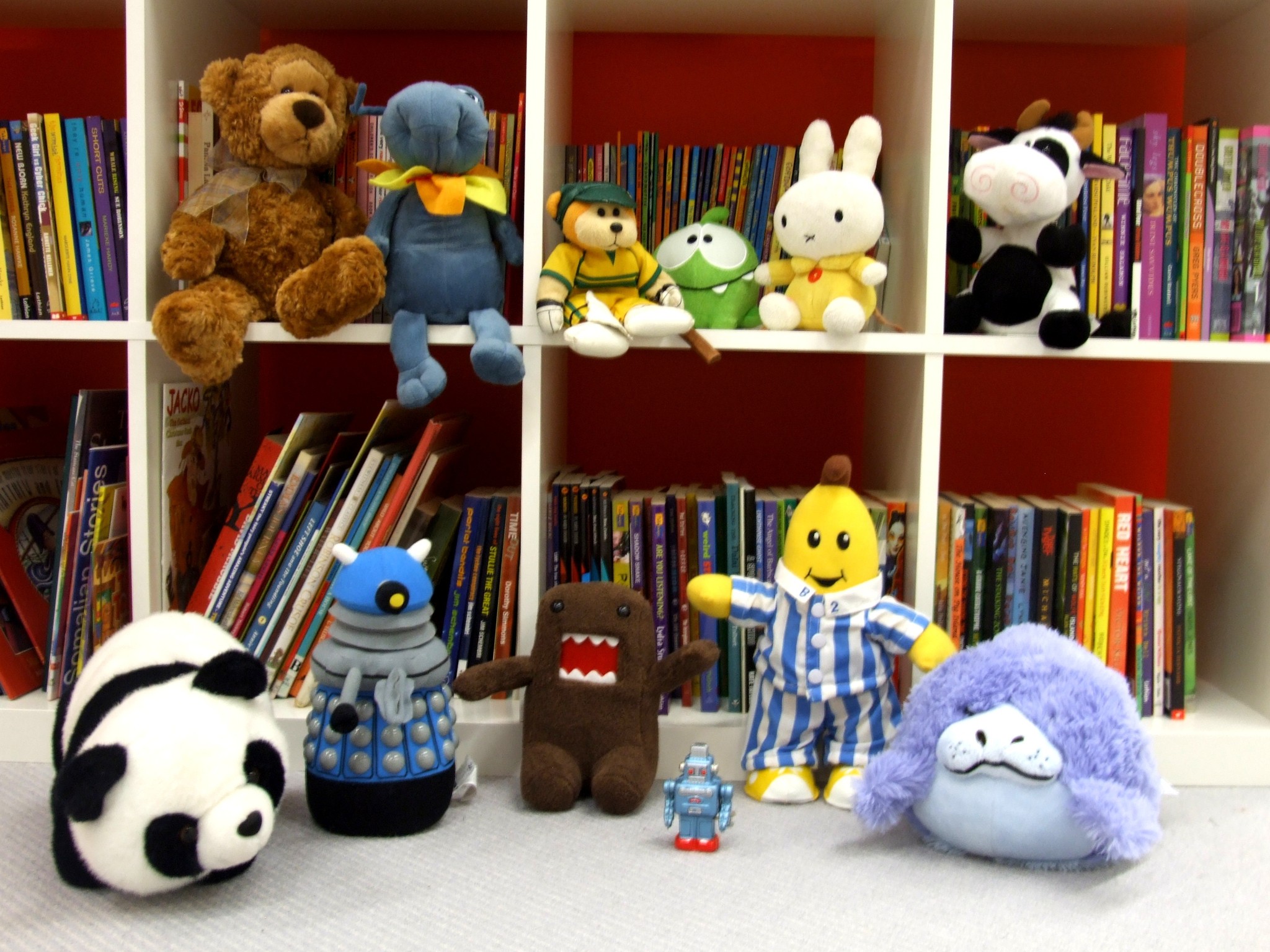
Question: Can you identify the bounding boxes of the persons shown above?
[1142,178,1163,218]
[662,742,733,852]
[886,510,907,578]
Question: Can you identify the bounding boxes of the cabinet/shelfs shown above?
[0,1,1270,792]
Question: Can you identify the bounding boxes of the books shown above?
[949,114,1270,341]
[0,112,127,321]
[0,386,129,700]
[177,80,525,328]
[160,379,519,707]
[936,482,1195,720]
[545,467,906,715]
[564,131,891,332]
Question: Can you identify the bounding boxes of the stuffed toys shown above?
[652,206,761,328]
[754,115,887,333]
[50,610,287,897]
[945,100,1126,350]
[854,623,1170,867]
[685,456,957,809]
[303,538,459,836]
[152,45,388,390]
[451,582,720,813]
[350,81,526,410]
[535,181,695,358]
